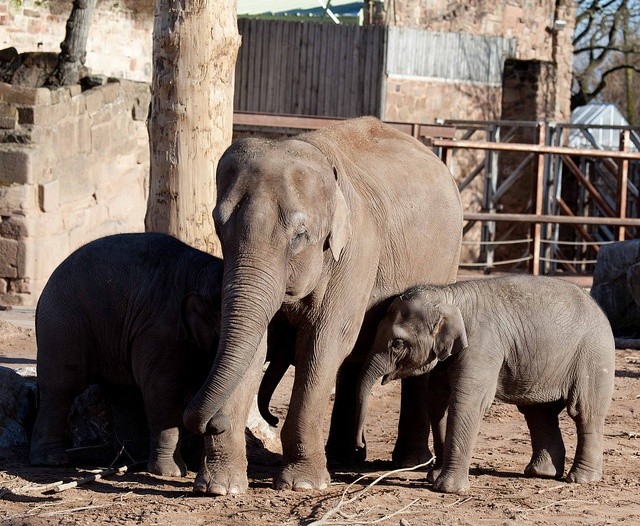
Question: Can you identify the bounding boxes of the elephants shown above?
[181,114,464,497]
[350,272,617,496]
[28,231,302,479]
